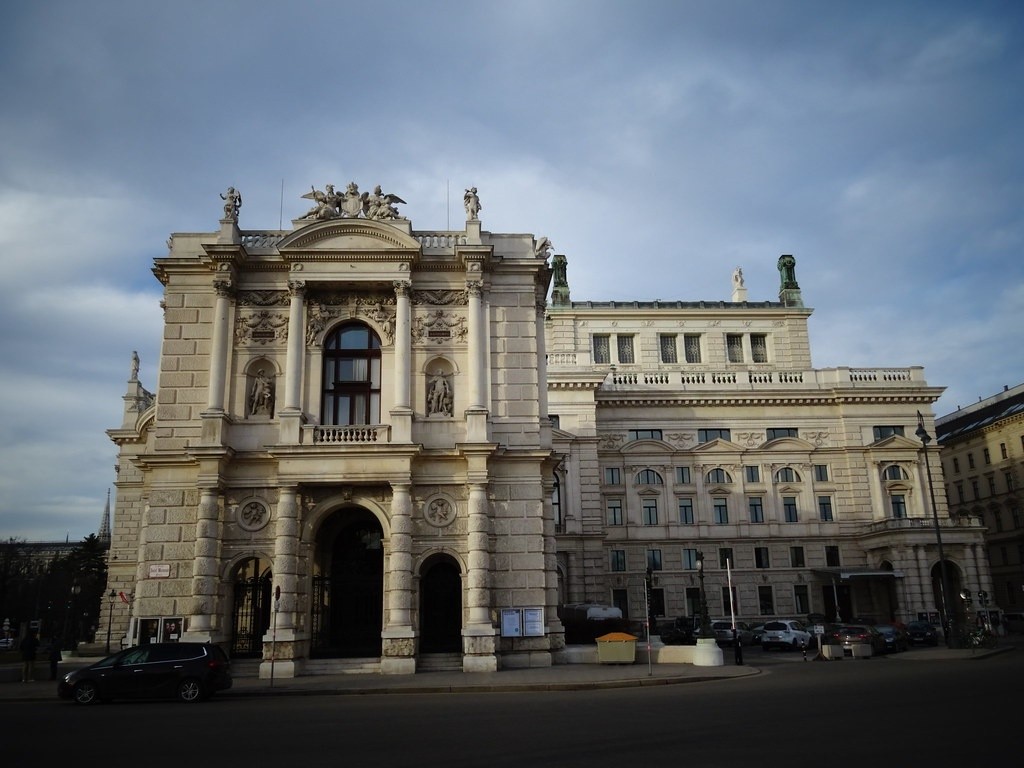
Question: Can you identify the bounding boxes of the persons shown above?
[249,369,271,414]
[428,370,449,413]
[314,185,349,209]
[463,187,482,220]
[131,351,139,382]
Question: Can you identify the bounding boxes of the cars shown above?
[873,625,908,652]
[57,641,232,705]
[0,638,16,650]
[710,621,755,644]
[831,625,890,655]
[903,621,940,646]
[761,620,814,652]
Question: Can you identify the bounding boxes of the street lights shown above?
[106,589,117,655]
[915,408,950,646]
[645,568,654,635]
[72,577,82,640]
[695,551,714,638]
